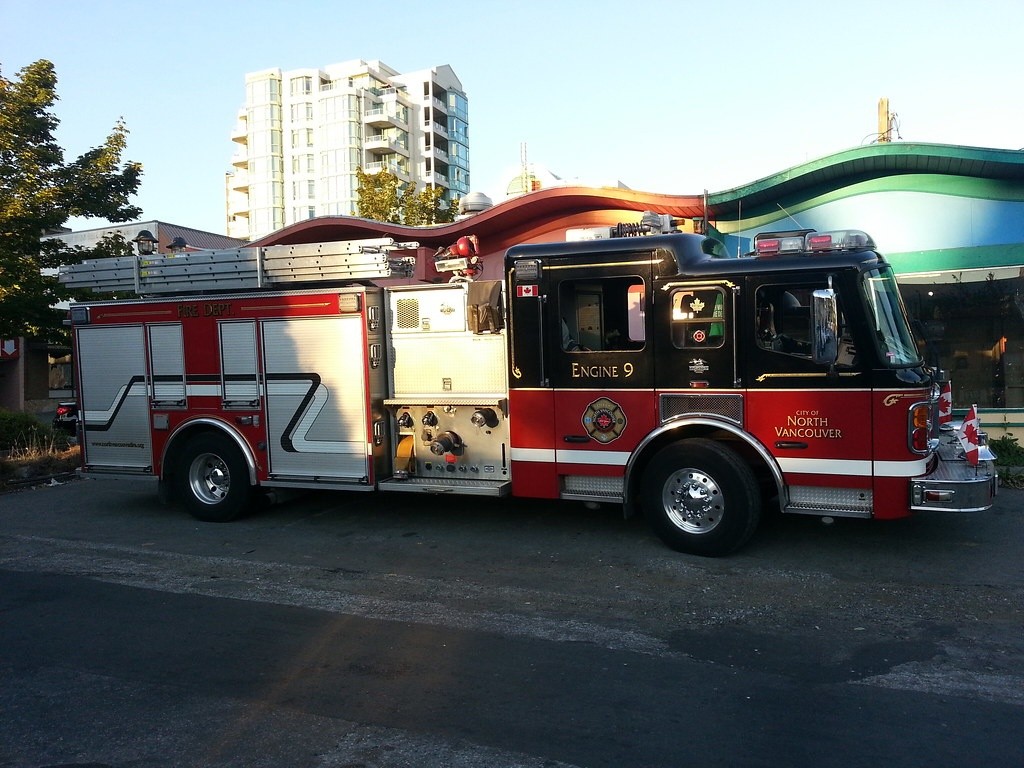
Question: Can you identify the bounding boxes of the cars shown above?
[52,401,78,438]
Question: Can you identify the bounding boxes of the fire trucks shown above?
[58,209,1001,560]
